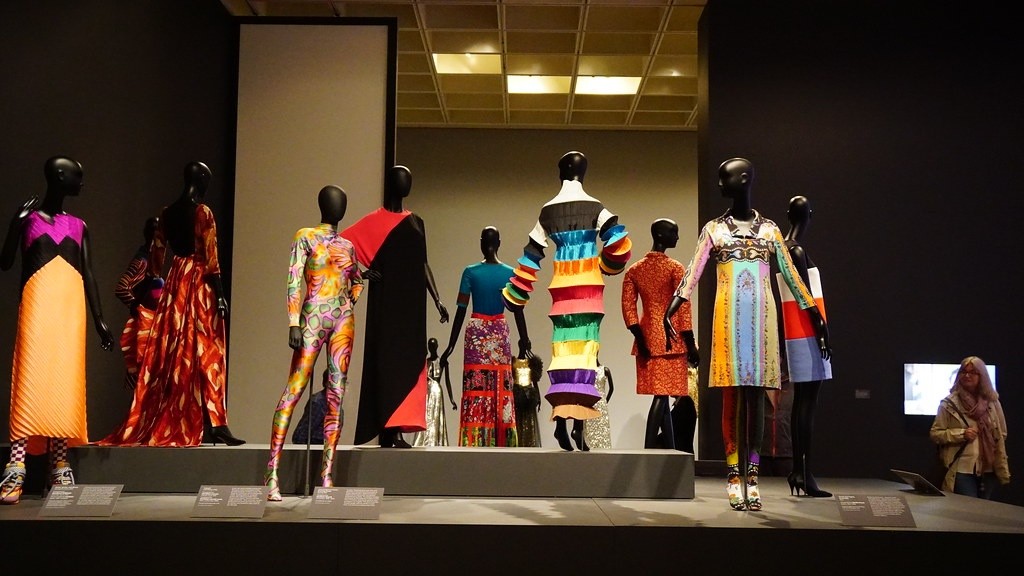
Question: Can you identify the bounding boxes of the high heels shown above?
[727,471,744,511]
[795,474,833,497]
[211,426,246,446]
[787,472,796,495]
[746,469,762,511]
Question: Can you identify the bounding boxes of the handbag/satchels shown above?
[935,466,948,491]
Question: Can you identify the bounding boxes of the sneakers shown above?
[47,463,75,491]
[0,463,26,505]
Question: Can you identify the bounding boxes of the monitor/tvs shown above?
[904,364,996,416]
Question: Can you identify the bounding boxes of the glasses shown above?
[959,370,980,376]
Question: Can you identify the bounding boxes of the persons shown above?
[263,186,364,504]
[511,338,542,448]
[292,368,344,446]
[770,195,832,497]
[930,356,1011,500]
[503,150,632,453]
[622,219,698,450]
[0,156,113,505]
[412,337,457,447]
[438,227,530,448]
[337,166,449,448]
[96,162,247,449]
[665,157,835,512]
